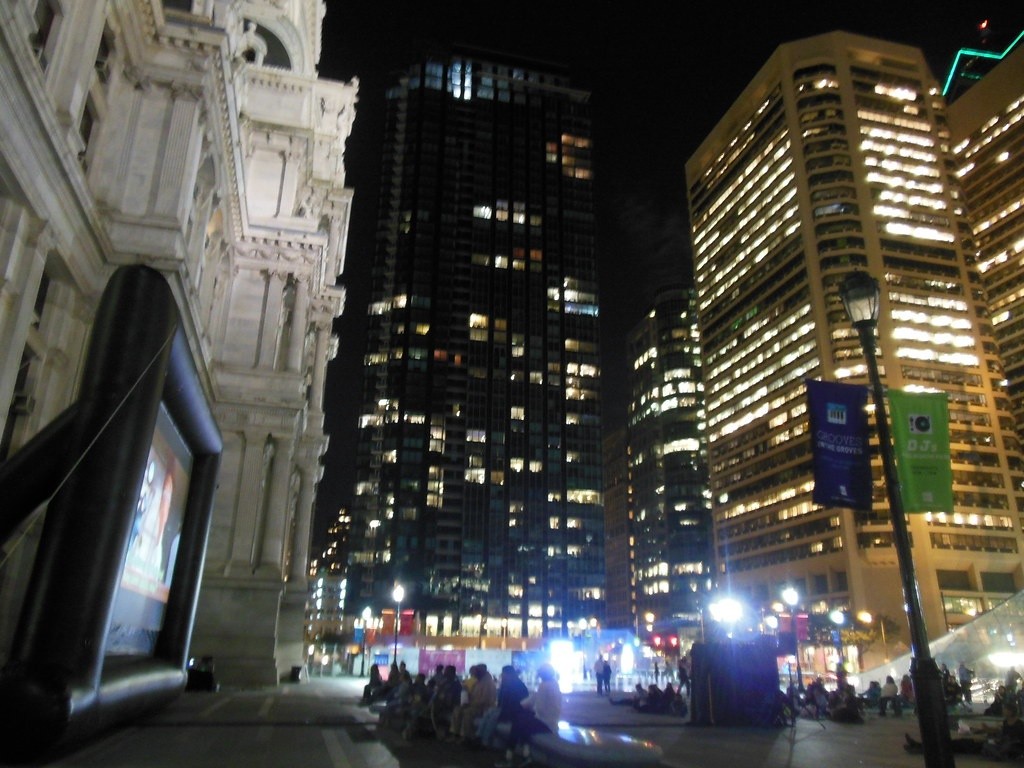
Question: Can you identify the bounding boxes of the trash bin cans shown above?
[290,666,302,685]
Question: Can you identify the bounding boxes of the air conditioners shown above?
[33,47,49,72]
[93,59,111,85]
[78,155,89,175]
[10,393,37,416]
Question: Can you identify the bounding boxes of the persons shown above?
[901,733,1009,753]
[984,686,1024,718]
[608,657,689,718]
[380,664,560,767]
[997,703,1024,759]
[603,661,612,693]
[788,661,975,720]
[879,674,901,716]
[360,664,381,704]
[595,656,604,695]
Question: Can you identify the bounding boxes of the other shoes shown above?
[518,757,531,768]
[456,735,472,746]
[494,759,512,767]
[471,744,486,751]
[905,734,920,749]
[469,737,481,744]
[904,744,917,755]
[443,732,460,744]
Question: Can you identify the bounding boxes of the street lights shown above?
[836,266,959,768]
[391,585,405,664]
[360,607,372,679]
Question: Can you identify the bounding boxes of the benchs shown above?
[368,702,665,768]
